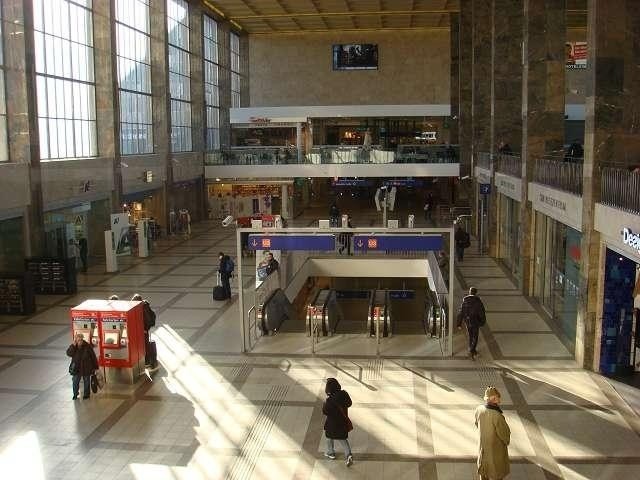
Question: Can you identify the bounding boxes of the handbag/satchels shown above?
[345,417,353,432]
[91,368,104,393]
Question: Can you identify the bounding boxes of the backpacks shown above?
[225,258,234,273]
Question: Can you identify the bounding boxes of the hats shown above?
[484,387,498,396]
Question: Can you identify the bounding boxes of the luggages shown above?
[147,329,157,364]
[213,270,225,300]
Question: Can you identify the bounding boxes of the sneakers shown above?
[346,455,352,467]
[325,452,335,459]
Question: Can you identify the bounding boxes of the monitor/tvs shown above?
[104,332,118,345]
[331,43,379,71]
[77,333,89,342]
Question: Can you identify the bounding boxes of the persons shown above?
[565,139,585,163]
[565,44,576,66]
[258,250,270,281]
[329,205,340,227]
[387,138,458,163]
[76,233,89,271]
[65,334,100,400]
[338,216,353,256]
[474,387,511,480]
[131,294,150,367]
[457,286,485,362]
[498,141,514,156]
[454,228,464,262]
[217,252,232,299]
[423,193,433,220]
[322,377,353,467]
[67,240,77,268]
[266,252,279,277]
[241,216,290,259]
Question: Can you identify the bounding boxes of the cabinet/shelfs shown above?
[0,271,37,316]
[23,257,77,296]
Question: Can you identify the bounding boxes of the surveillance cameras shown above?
[221,215,233,227]
